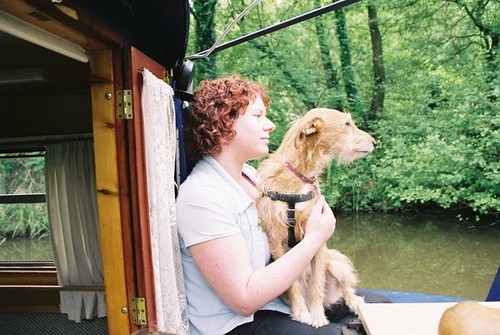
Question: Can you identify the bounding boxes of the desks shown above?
[356,299,500,335]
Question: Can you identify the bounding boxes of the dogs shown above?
[254,106,377,330]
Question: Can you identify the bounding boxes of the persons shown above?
[174,75,367,335]
